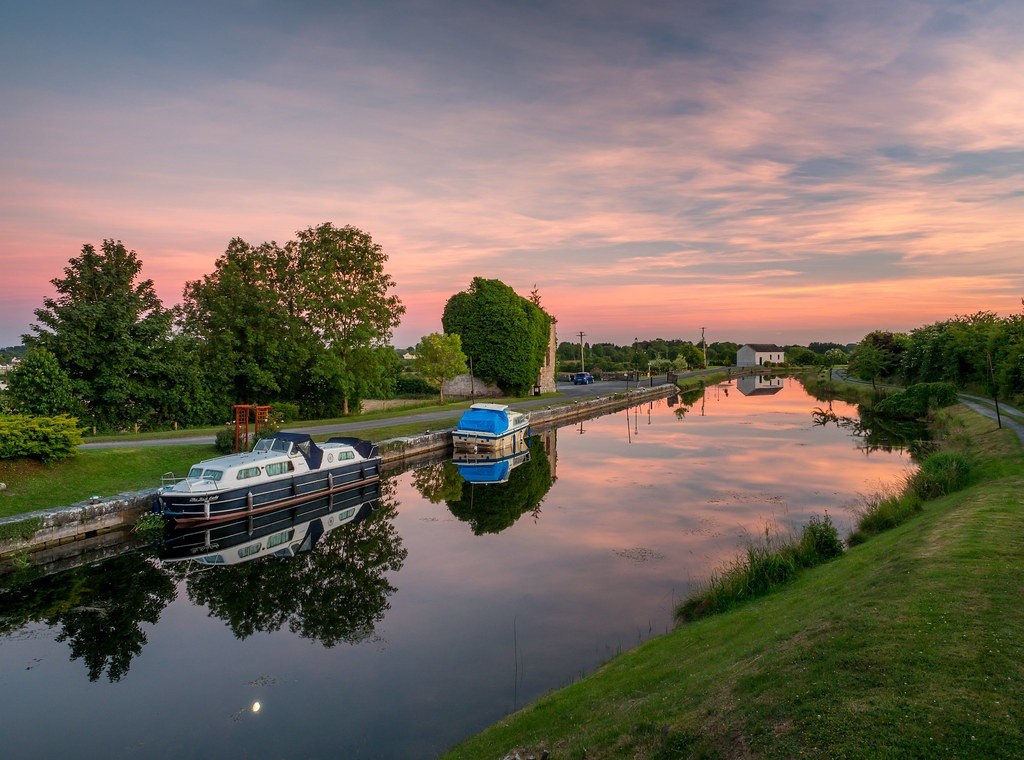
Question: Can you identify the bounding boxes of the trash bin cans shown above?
[533,385,541,396]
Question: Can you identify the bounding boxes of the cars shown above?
[573,372,594,385]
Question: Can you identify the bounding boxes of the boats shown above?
[451,403,531,451]
[155,480,382,578]
[157,432,382,524]
[451,439,532,485]
